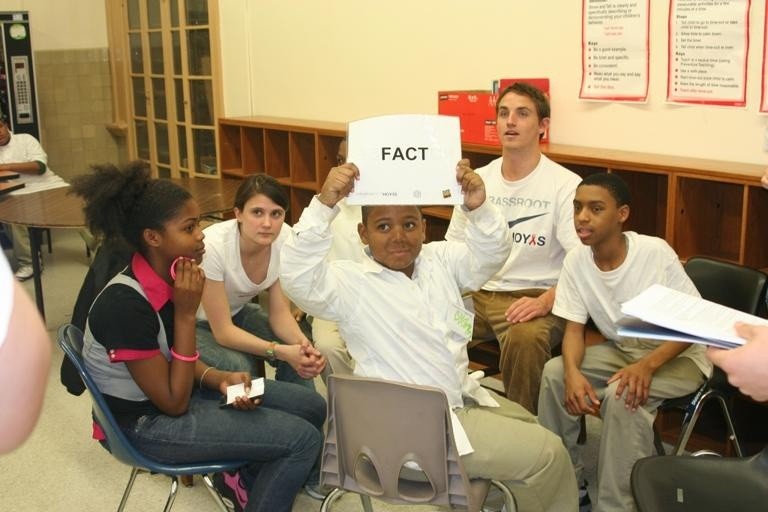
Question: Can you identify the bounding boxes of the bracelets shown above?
[265,340,278,362]
[197,365,216,392]
[169,346,200,363]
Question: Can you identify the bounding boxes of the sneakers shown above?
[305,478,343,501]
[212,468,250,511]
[14,265,41,281]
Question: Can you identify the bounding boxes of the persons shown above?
[274,159,582,510]
[1,245,53,457]
[535,172,715,510]
[438,81,583,421]
[184,172,339,502]
[0,119,102,283]
[66,159,328,512]
[706,321,768,404]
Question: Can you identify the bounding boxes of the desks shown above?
[0,173,245,330]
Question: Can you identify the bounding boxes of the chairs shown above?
[318,374,517,512]
[593,258,768,458]
[58,322,250,512]
[469,338,587,445]
[629,455,767,512]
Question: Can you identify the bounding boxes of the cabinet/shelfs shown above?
[213,112,767,460]
